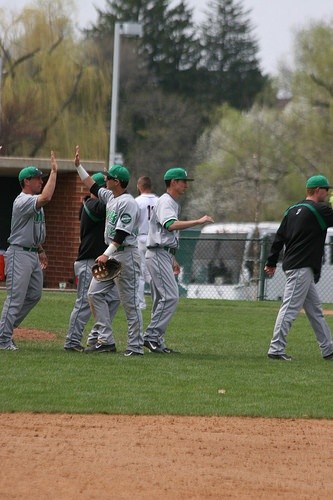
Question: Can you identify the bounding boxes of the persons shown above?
[72,145,147,357]
[265,174,333,362]
[140,168,215,352]
[62,172,121,352]
[0,151,58,351]
[127,173,168,308]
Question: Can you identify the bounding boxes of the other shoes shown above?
[2,343,18,350]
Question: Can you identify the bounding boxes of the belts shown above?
[164,247,175,255]
[23,247,38,252]
[117,246,124,251]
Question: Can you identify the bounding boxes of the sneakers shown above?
[68,345,85,352]
[163,348,177,354]
[268,353,292,361]
[84,342,115,353]
[118,350,142,357]
[324,353,333,359]
[143,340,163,353]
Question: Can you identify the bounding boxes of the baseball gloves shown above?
[91,258,122,282]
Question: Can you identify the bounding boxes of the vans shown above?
[185,221,333,304]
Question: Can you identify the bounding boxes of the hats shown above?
[18,166,48,181]
[305,175,333,188]
[164,168,195,181]
[91,173,106,186]
[104,165,129,180]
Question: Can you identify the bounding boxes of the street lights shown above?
[108,20,143,168]
